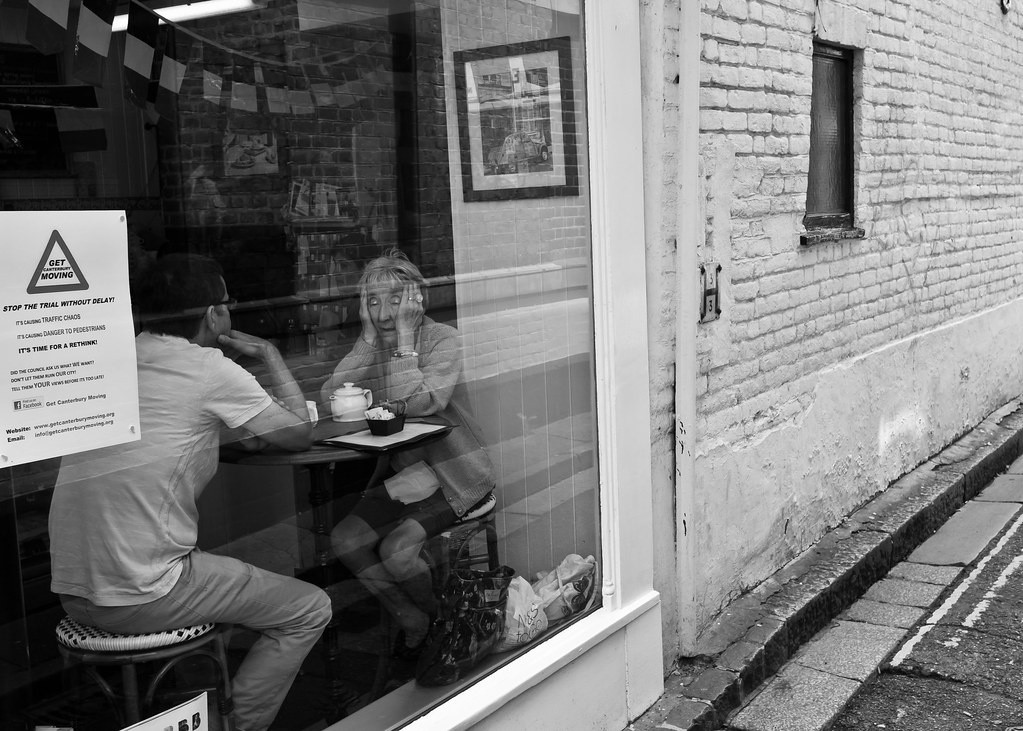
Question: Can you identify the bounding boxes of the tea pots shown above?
[329,382,373,423]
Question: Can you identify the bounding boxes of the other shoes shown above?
[386,611,436,681]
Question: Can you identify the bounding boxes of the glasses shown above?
[201,299,237,316]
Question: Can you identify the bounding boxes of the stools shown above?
[57,615,234,731]
[390,493,501,654]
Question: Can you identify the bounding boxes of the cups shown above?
[305,400,318,429]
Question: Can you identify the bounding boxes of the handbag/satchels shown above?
[493,575,549,654]
[530,554,600,628]
[415,524,517,687]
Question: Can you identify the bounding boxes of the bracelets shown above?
[395,350,419,357]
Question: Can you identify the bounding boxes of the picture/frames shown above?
[453,36,580,202]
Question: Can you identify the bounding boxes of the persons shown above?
[322,252,495,683]
[47,253,334,731]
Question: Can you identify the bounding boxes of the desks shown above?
[218,414,454,724]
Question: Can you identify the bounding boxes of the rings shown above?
[415,294,423,303]
[408,297,414,301]
